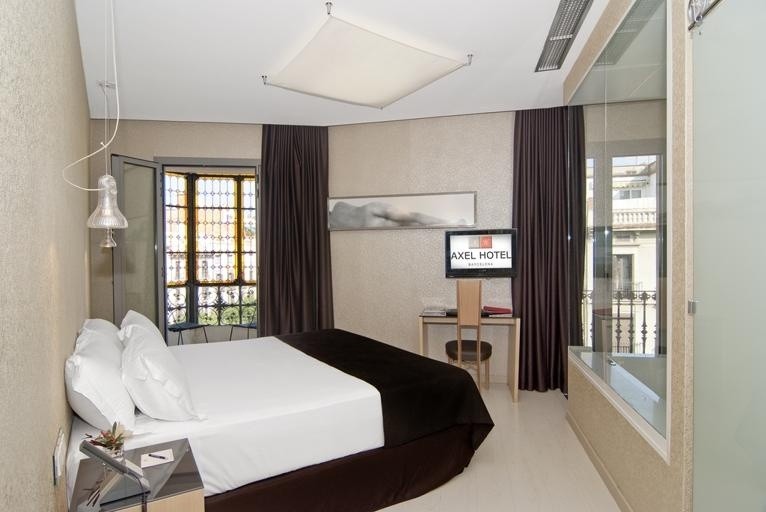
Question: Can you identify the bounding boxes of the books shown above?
[421,305,512,317]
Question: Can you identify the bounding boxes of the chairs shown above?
[168,305,209,345]
[446,281,492,393]
[229,306,257,340]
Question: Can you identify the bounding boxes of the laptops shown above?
[446,309,489,317]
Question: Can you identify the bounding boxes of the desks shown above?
[419,316,520,403]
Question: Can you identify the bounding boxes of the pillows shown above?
[64,309,205,432]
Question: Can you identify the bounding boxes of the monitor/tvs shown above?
[445,228,518,279]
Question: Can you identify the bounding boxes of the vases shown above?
[102,441,124,465]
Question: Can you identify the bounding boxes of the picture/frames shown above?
[327,191,477,231]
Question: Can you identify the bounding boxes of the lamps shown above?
[61,0,128,249]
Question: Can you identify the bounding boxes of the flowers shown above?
[83,422,134,454]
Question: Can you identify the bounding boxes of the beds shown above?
[67,328,495,512]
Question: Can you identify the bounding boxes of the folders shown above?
[484,307,511,314]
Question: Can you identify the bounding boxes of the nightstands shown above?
[69,438,205,512]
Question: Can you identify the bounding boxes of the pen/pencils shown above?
[149,454,165,459]
